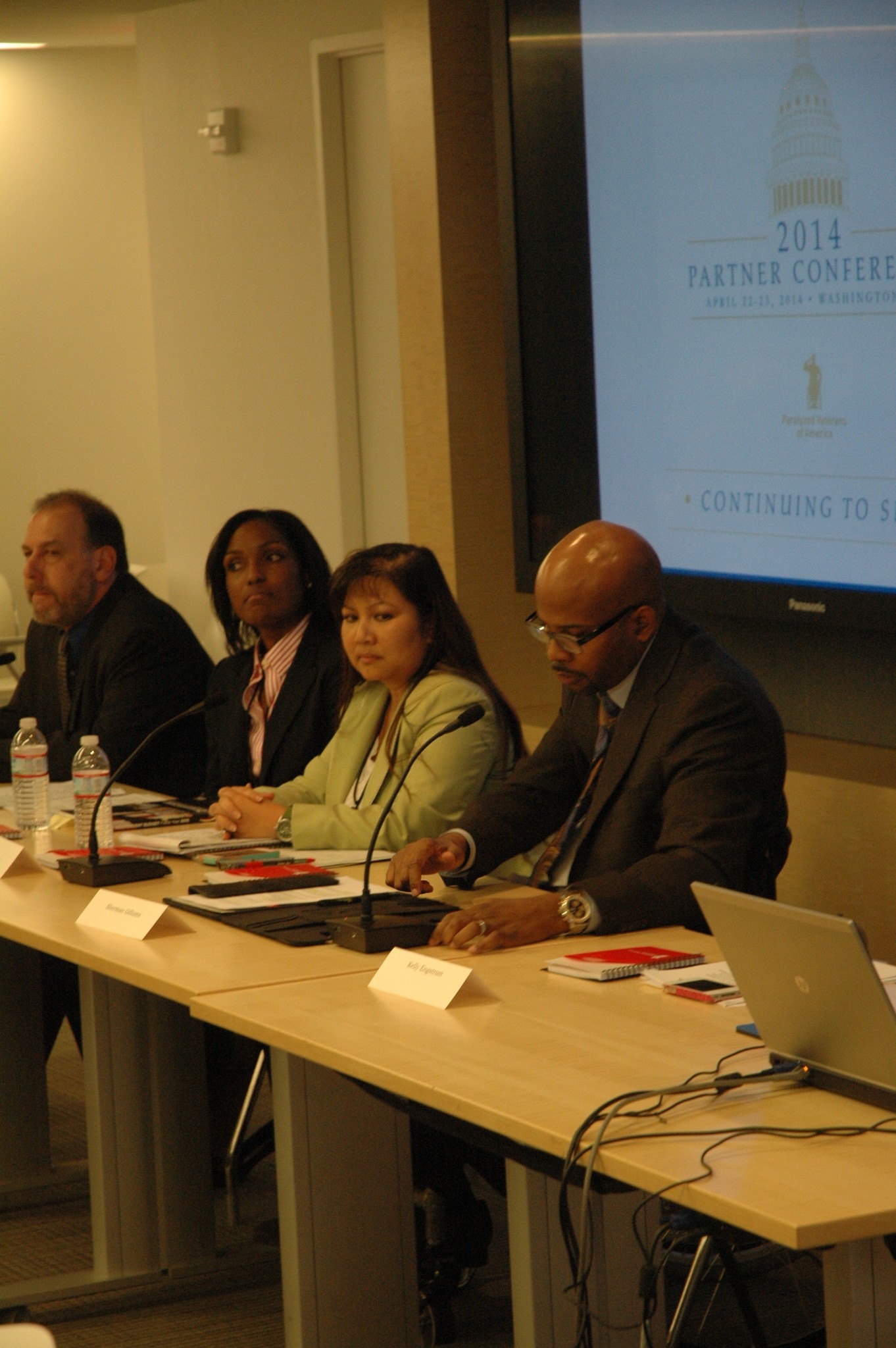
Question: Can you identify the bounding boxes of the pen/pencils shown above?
[235,857,315,868]
[206,852,280,865]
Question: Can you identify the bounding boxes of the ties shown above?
[529,692,622,890]
[57,633,73,732]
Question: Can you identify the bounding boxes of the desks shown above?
[0,780,896,1348]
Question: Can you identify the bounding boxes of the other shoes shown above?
[214,1119,274,1186]
[418,1199,494,1281]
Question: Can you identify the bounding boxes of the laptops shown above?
[689,879,896,1112]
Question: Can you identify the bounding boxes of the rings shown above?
[476,920,490,937]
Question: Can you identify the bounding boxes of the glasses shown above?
[522,598,653,654]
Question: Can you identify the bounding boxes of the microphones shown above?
[56,690,230,888]
[322,704,488,953]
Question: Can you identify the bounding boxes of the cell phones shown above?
[663,978,743,1004]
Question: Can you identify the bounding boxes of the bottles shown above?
[11,717,50,832]
[72,736,113,850]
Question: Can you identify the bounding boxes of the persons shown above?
[0,491,216,1063]
[193,510,793,1289]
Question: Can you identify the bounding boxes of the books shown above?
[546,946,706,982]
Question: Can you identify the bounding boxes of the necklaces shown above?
[353,697,397,810]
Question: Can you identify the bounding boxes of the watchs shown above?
[558,890,591,937]
[274,803,294,842]
